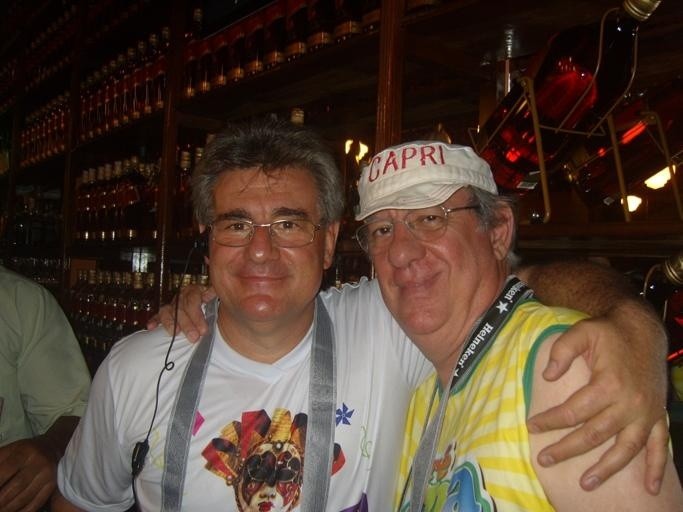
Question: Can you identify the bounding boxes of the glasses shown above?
[208,218,328,248]
[351,204,482,256]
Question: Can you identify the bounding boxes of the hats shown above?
[353,139,500,221]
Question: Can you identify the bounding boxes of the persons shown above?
[51,116,667,512]
[1,262,92,512]
[144,141,682,512]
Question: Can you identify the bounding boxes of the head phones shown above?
[191,224,210,258]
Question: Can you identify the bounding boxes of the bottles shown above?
[629,255,682,409]
[0,1,450,358]
[455,1,663,212]
